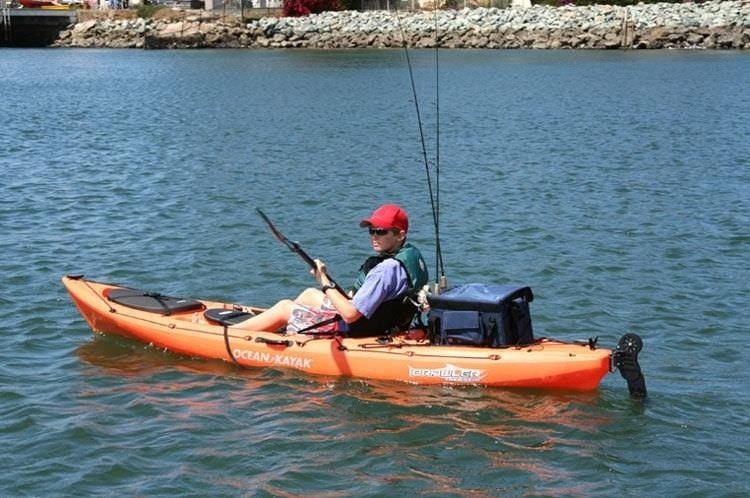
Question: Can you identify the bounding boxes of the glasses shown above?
[368,227,399,236]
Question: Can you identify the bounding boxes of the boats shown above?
[0,0,81,51]
[58,266,650,401]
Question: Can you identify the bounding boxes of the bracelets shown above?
[321,281,337,294]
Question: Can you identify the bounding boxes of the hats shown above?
[359,204,409,234]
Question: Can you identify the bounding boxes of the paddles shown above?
[257,208,348,297]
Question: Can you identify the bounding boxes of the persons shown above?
[190,204,431,337]
